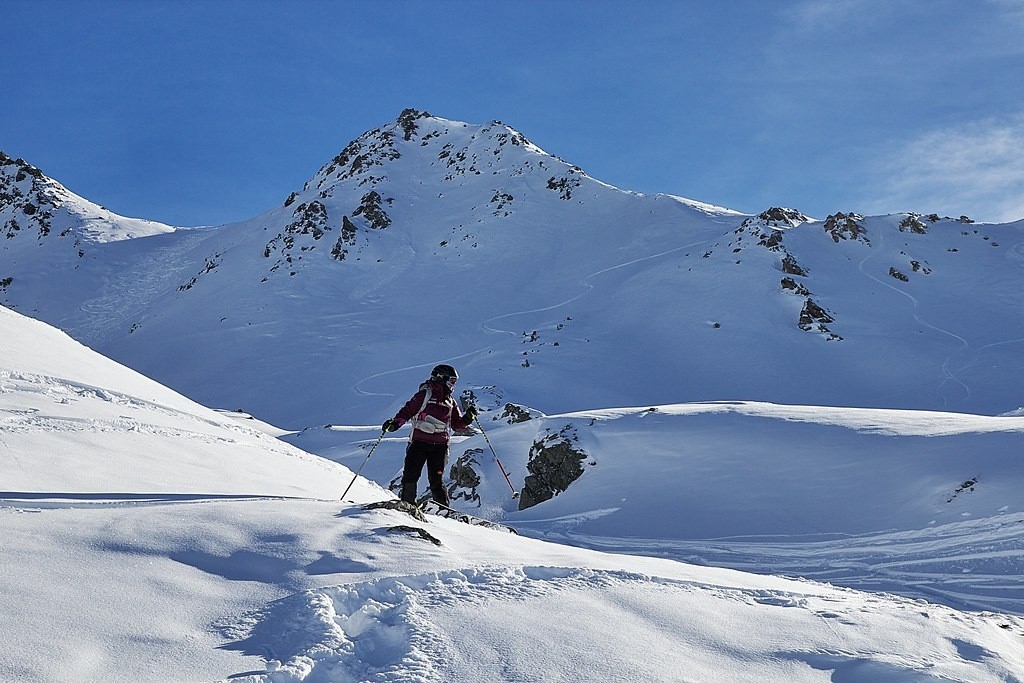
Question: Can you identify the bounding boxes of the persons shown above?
[381,364,479,507]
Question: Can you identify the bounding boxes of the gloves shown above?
[382,417,399,433]
[461,405,477,425]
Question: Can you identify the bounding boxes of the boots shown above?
[400,482,416,506]
[430,482,450,509]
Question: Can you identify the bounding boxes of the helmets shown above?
[430,364,459,379]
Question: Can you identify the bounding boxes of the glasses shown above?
[447,377,457,384]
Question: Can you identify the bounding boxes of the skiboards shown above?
[417,500,518,536]
